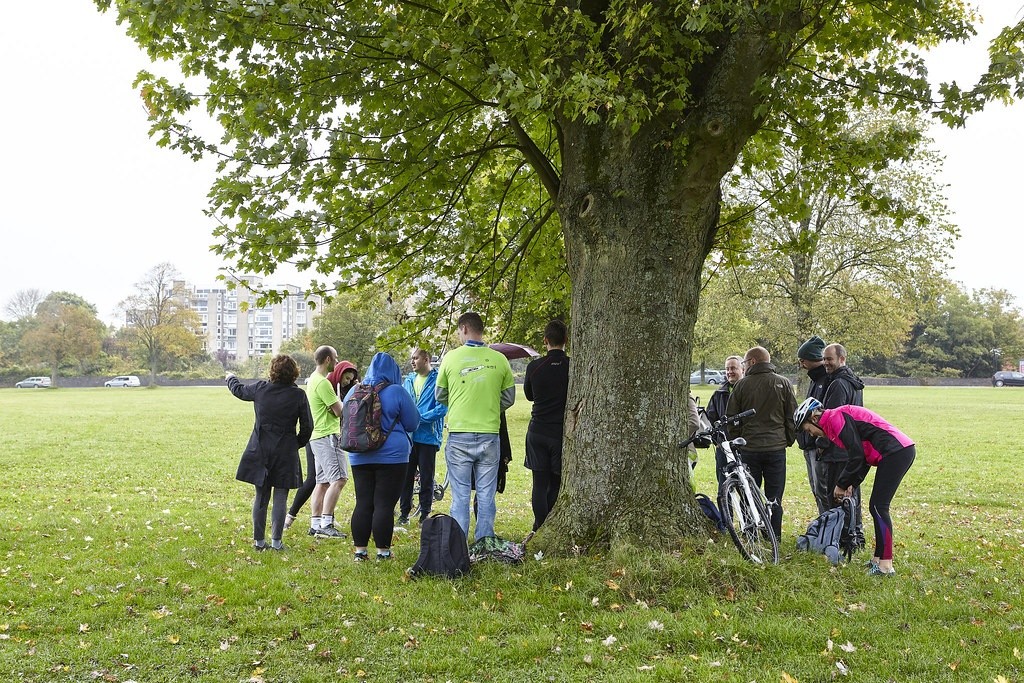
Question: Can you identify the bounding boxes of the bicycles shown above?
[393,426,449,520]
[679,409,779,568]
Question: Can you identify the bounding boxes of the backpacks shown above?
[689,396,713,448]
[409,513,471,583]
[797,496,857,567]
[339,381,400,452]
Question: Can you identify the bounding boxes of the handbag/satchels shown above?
[695,493,727,536]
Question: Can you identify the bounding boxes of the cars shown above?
[719,370,728,383]
[690,370,724,384]
[992,371,1024,387]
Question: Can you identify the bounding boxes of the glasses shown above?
[740,358,753,367]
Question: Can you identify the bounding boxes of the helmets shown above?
[793,396,823,430]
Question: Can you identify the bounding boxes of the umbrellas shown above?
[488,343,541,360]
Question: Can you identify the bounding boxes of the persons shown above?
[225,353,315,553]
[284,346,359,540]
[398,349,449,526]
[706,355,747,532]
[523,320,575,531]
[342,352,422,563]
[795,336,866,553]
[723,346,800,546]
[686,394,697,479]
[434,313,515,545]
[793,396,916,578]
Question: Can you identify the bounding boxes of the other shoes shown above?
[417,515,430,527]
[334,520,345,528]
[396,514,410,526]
[282,522,290,531]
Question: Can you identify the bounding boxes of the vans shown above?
[104,376,140,387]
[15,377,51,388]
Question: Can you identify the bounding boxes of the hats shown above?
[797,335,827,361]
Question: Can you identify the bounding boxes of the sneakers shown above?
[354,553,369,561]
[255,542,271,550]
[867,559,874,567]
[315,524,347,539]
[868,565,896,577]
[270,542,284,551]
[376,550,393,562]
[306,527,315,538]
